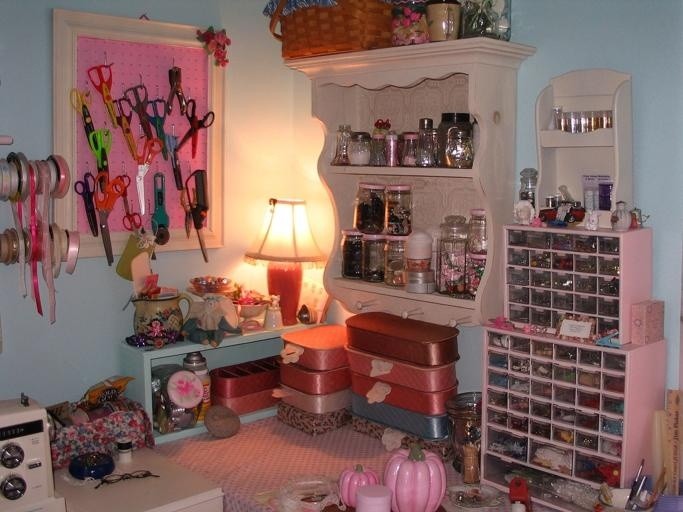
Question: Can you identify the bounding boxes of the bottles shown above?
[331,113,488,298]
[551,105,612,133]
[151,352,210,435]
[391,1,511,47]
[519,168,642,232]
[459,457,480,487]
[264,307,283,331]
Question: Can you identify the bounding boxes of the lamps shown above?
[245,198,326,325]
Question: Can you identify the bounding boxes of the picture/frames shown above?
[53,10,225,259]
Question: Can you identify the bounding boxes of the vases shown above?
[284,36,536,329]
[388,5,428,44]
[425,3,460,41]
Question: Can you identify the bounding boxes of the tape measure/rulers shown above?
[667,389,683,496]
[653,410,668,496]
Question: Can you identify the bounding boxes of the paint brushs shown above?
[601,459,668,511]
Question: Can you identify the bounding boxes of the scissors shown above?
[70,63,215,267]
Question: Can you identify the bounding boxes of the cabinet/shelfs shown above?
[122,324,310,446]
[479,68,667,512]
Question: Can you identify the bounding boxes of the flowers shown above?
[194,25,230,67]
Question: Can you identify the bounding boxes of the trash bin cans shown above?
[444,392,482,473]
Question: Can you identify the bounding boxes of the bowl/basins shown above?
[190,276,232,293]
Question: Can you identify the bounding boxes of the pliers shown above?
[165,66,187,115]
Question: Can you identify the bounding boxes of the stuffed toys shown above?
[177,291,244,347]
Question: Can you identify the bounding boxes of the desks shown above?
[54,447,226,512]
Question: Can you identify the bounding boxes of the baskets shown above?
[280,0,392,59]
